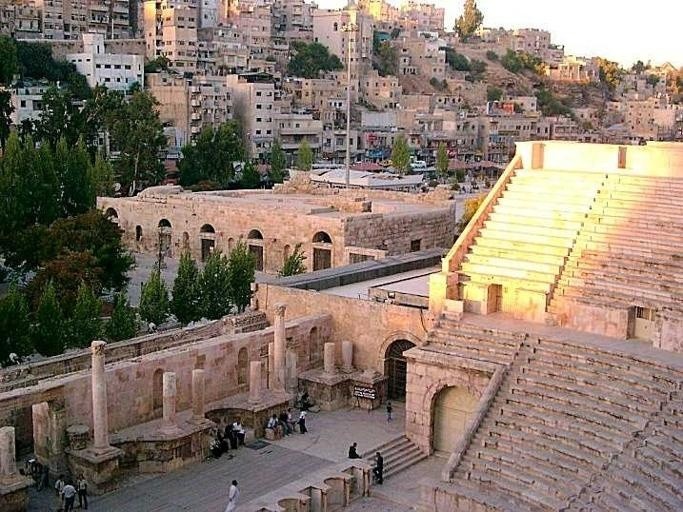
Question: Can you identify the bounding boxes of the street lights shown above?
[339,23,359,187]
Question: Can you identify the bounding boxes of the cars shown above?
[410,160,426,169]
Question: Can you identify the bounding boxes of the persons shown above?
[222,480,240,512]
[371,452,383,485]
[53,474,65,512]
[385,402,392,423]
[75,474,88,510]
[58,481,77,512]
[348,442,362,460]
[208,407,307,460]
